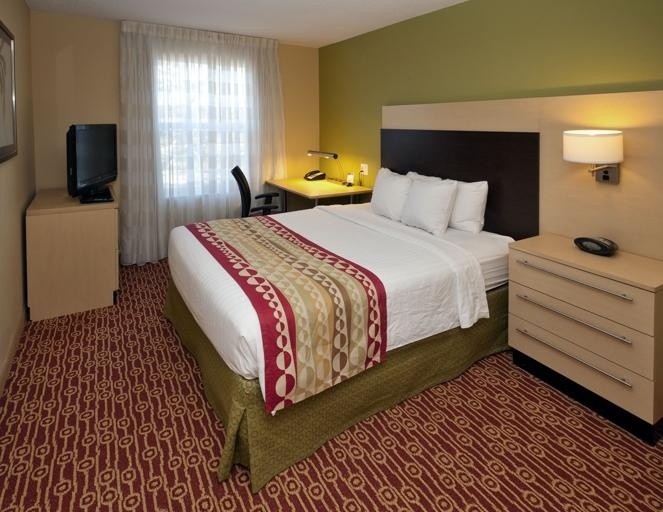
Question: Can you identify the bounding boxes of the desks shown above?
[265,176,373,211]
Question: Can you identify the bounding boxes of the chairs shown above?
[229,166,284,218]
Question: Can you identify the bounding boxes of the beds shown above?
[157,128,541,493]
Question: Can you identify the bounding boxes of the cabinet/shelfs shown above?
[23,184,119,321]
[507,230,663,446]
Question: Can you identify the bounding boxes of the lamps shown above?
[561,129,624,185]
[304,149,346,185]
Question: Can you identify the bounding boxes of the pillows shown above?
[442,179,488,234]
[367,167,413,221]
[399,179,458,237]
[404,170,442,182]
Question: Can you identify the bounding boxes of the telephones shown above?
[304,170,326,181]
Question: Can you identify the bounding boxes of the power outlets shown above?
[360,164,368,176]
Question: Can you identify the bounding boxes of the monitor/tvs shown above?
[66,124,117,203]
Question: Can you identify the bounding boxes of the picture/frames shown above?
[0,21,19,163]
